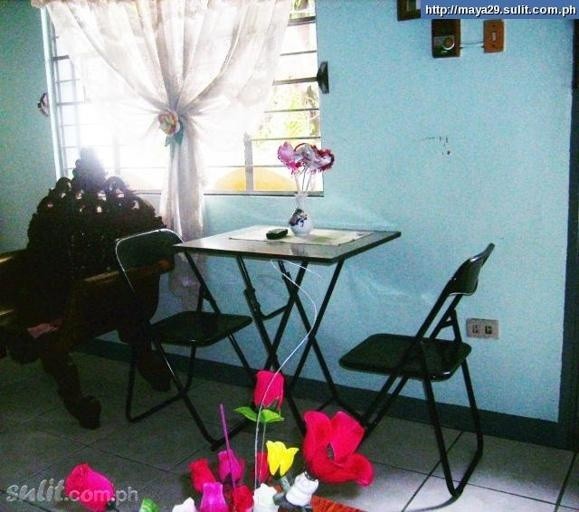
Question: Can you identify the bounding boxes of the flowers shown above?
[278,142,335,195]
[64,368,373,511]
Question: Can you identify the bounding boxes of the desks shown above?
[172,225,401,456]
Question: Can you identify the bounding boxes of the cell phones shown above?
[266,228,288,239]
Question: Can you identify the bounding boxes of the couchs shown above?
[1,148,183,429]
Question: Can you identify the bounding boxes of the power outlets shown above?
[466,318,498,339]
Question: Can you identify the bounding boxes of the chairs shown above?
[338,242,496,499]
[114,229,258,451]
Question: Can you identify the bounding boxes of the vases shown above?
[290,195,313,238]
